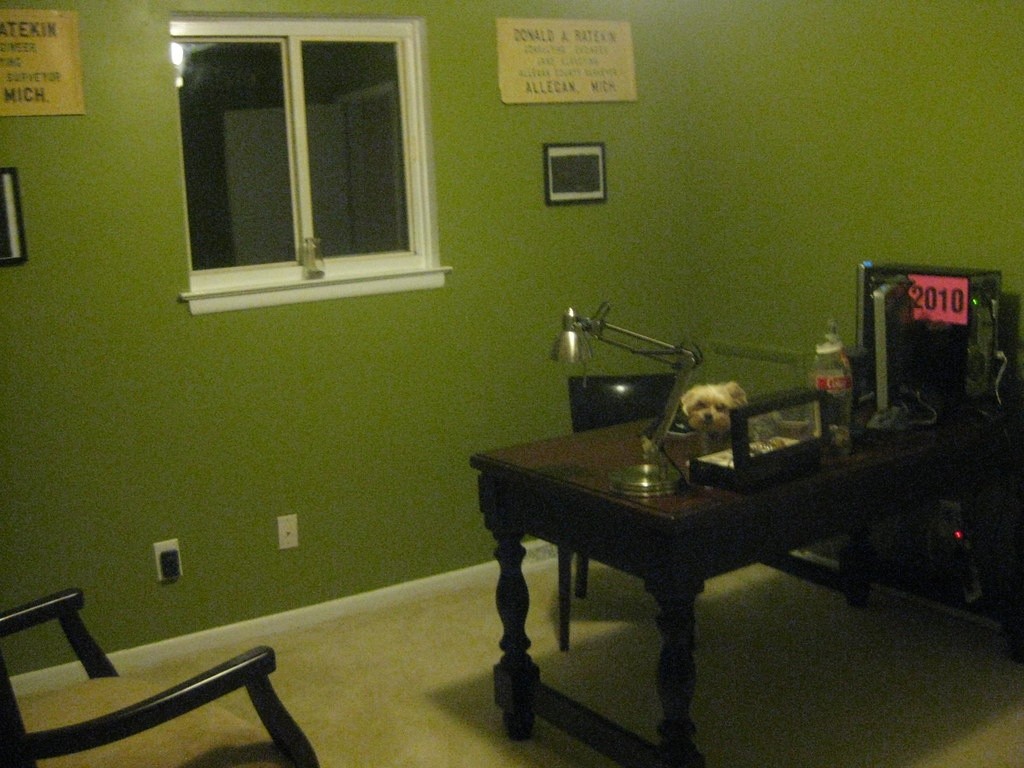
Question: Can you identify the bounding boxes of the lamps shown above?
[551,301,696,497]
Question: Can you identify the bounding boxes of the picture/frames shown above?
[0,167,28,267]
[545,142,607,205]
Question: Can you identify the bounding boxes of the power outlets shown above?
[153,538,184,582]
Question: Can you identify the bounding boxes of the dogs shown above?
[681,381,812,444]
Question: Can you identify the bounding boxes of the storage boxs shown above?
[690,388,830,494]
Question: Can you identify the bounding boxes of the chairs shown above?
[559,373,705,652]
[0,588,320,768]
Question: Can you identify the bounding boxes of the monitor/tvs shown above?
[864,275,944,431]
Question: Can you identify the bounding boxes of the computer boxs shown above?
[857,263,999,404]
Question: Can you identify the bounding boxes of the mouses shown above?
[652,415,696,439]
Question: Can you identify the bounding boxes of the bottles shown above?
[810,323,853,453]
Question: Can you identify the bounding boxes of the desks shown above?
[469,388,1024,768]
